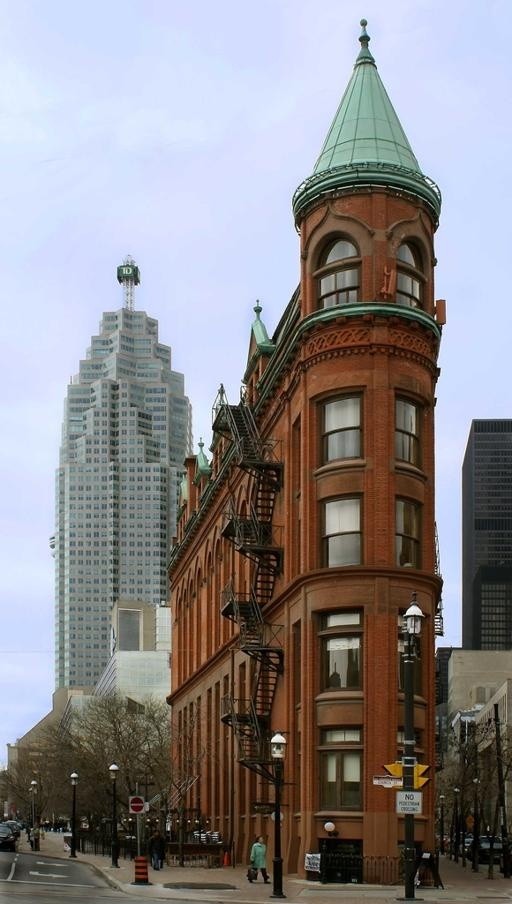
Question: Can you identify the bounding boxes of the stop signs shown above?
[129,797,145,813]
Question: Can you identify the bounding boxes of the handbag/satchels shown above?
[247,869,258,880]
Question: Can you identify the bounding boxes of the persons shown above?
[443,838,450,852]
[26,818,64,851]
[148,829,164,870]
[250,836,271,884]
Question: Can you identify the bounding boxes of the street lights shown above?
[108,765,120,866]
[439,795,446,855]
[70,771,78,857]
[28,781,38,829]
[472,778,482,872]
[403,595,425,898]
[269,733,286,898]
[453,788,460,862]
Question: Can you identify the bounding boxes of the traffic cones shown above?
[223,852,230,866]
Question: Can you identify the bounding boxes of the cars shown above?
[436,832,512,874]
[0,820,23,853]
